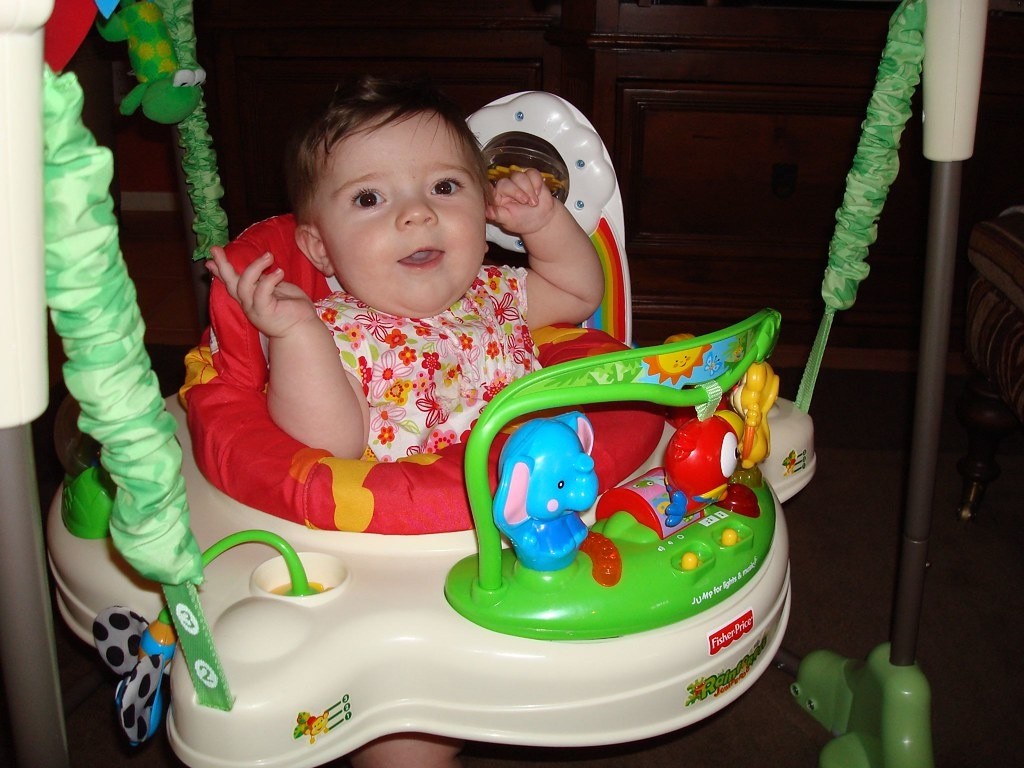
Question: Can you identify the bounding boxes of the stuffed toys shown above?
[95,0,205,122]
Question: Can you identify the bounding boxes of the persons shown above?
[206,78,606,768]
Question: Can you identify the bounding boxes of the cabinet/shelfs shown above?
[194,0,1024,378]
